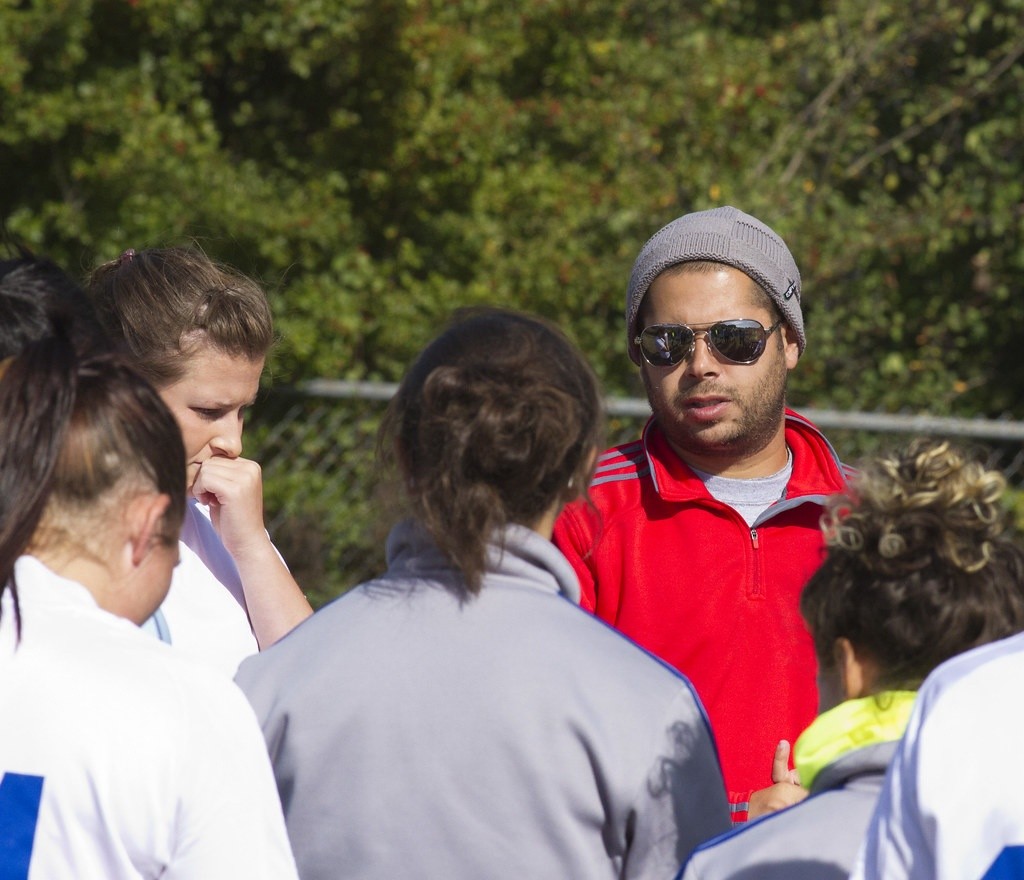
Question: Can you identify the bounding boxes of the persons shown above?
[544,205,883,832]
[228,305,734,880]
[1,255,111,369]
[0,337,299,880]
[81,243,318,682]
[850,630,1023,880]
[667,433,1022,880]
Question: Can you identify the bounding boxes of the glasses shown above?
[634,319,783,369]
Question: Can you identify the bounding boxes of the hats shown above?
[626,205,806,366]
